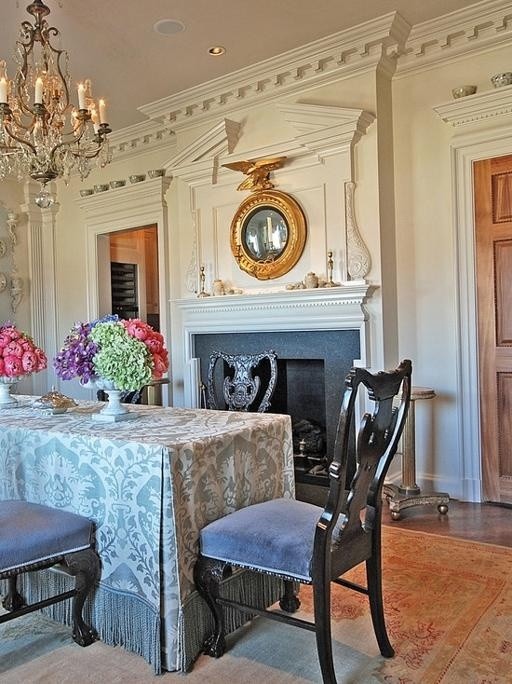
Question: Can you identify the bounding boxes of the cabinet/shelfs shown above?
[109,260,137,320]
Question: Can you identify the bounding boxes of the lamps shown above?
[1,2,113,208]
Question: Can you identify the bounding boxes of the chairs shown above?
[196,361,414,678]
[3,499,99,654]
[204,350,280,413]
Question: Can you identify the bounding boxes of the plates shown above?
[77,400,105,414]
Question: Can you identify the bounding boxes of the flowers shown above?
[0,312,169,393]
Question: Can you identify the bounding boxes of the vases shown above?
[80,375,144,421]
[1,374,27,408]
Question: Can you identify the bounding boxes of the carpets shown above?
[1,521,511,681]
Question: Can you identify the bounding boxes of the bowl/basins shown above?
[451,85,477,99]
[490,72,512,89]
[79,169,165,197]
